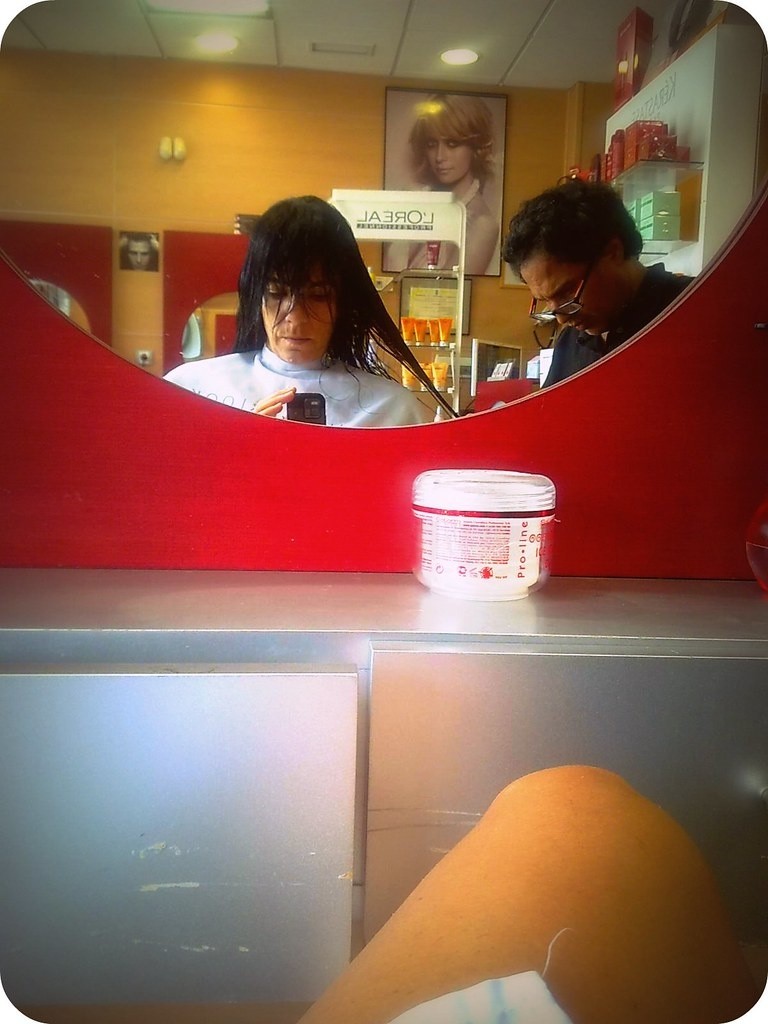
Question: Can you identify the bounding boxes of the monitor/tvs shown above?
[471,338,522,396]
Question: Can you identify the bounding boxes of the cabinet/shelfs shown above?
[605,22,762,273]
[329,185,470,418]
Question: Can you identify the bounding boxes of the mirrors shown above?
[0,0,768,431]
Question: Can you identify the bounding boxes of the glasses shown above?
[528,260,603,321]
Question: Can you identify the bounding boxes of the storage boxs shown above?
[567,0,756,259]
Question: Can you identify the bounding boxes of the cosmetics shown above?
[394,315,453,392]
[408,470,558,601]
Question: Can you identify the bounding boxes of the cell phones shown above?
[286,392,327,426]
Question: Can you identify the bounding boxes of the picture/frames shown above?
[380,85,529,339]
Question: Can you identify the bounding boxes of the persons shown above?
[383,93,501,274]
[501,175,695,389]
[163,195,424,427]
[298,763,758,1024]
[119,233,159,271]
